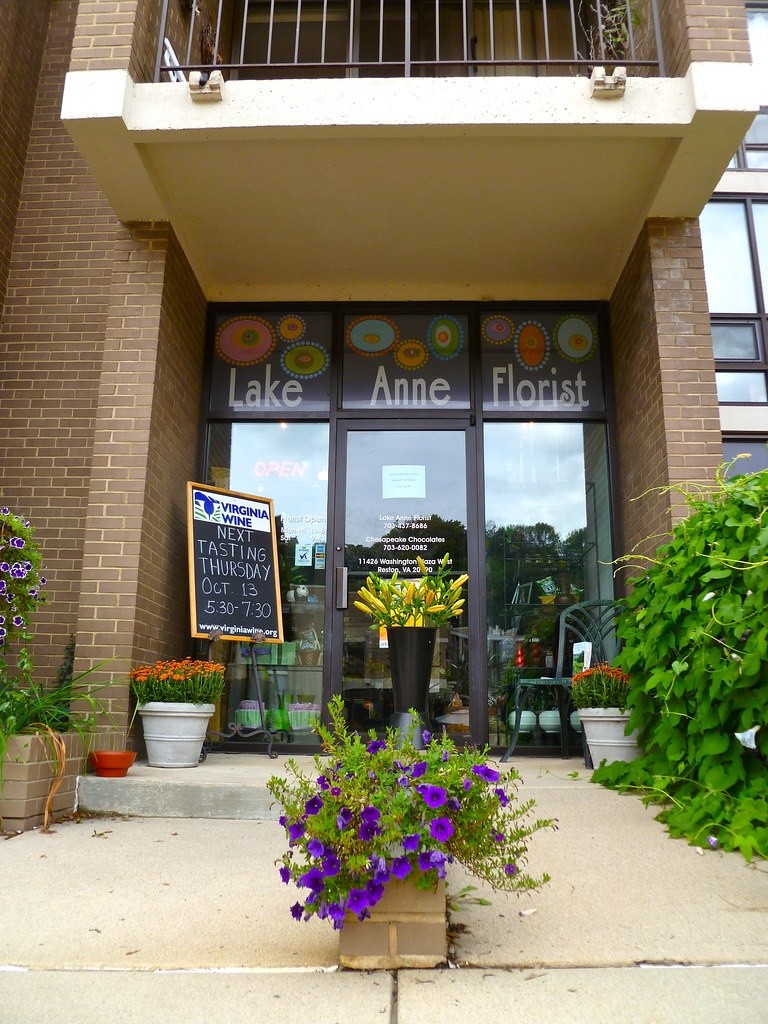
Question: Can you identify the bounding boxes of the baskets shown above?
[296,626,323,666]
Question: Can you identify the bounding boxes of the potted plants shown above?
[507,688,538,733]
[0,654,132,836]
[536,685,561,734]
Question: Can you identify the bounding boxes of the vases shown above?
[137,701,217,769]
[383,625,442,750]
[570,711,583,733]
[578,705,646,771]
[335,856,449,971]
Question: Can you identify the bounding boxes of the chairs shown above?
[498,598,630,771]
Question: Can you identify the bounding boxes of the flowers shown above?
[263,692,559,933]
[0,507,52,648]
[128,656,227,706]
[566,660,634,708]
[353,551,469,629]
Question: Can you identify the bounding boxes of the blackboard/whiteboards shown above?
[184,480,285,646]
